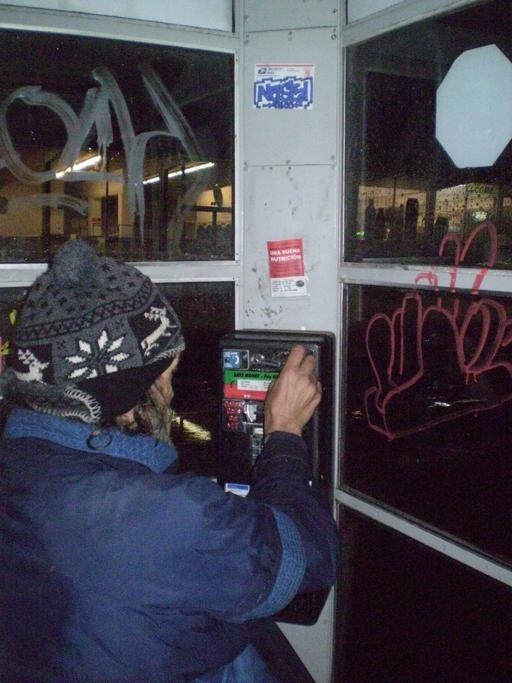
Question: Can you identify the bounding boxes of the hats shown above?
[10,238,187,424]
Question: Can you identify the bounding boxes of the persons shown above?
[0,235,342,683]
